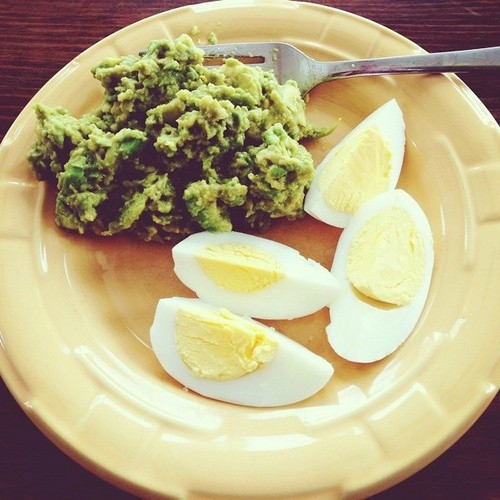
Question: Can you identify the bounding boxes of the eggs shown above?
[324,184,436,367]
[171,227,341,322]
[302,97,409,229]
[150,296,336,412]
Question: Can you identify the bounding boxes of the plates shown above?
[0,0,499,500]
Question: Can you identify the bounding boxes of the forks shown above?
[190,39,499,100]
